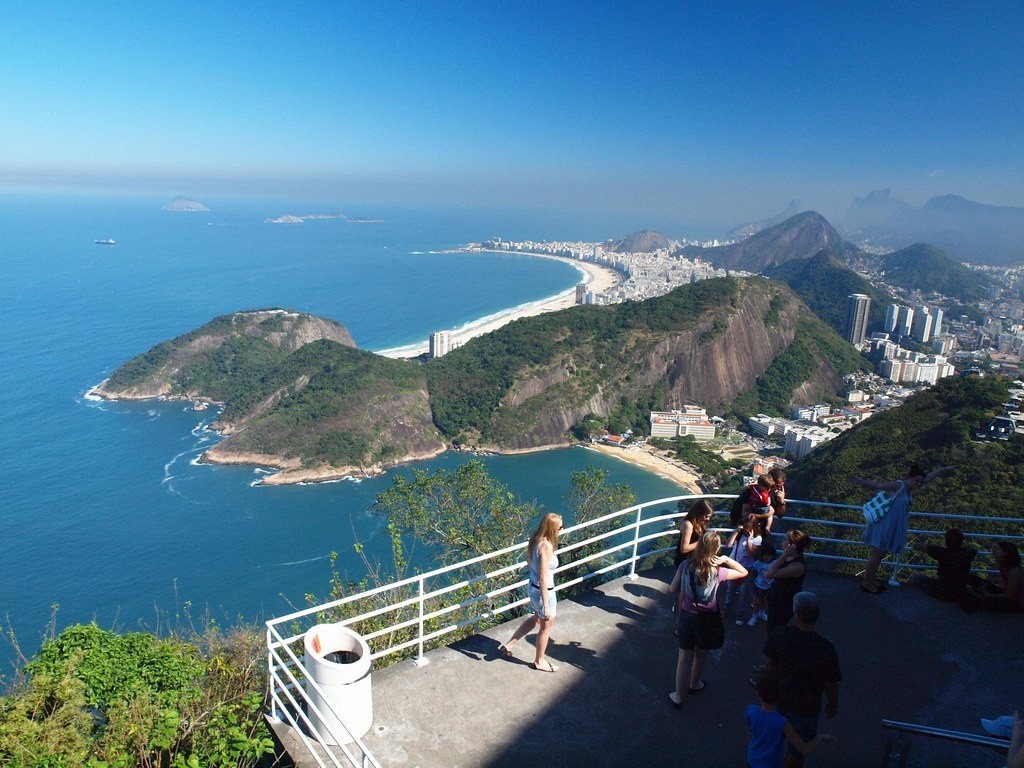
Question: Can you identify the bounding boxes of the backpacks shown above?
[730,496,743,527]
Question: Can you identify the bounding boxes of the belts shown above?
[529,581,553,590]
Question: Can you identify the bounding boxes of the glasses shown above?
[558,526,564,531]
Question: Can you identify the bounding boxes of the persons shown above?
[667,530,749,707]
[673,499,714,638]
[721,469,786,628]
[497,513,564,672]
[750,530,811,686]
[844,467,953,594]
[759,591,842,768]
[912,529,1024,614]
[744,663,838,768]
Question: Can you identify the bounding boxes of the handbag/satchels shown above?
[695,609,725,649]
[863,480,904,523]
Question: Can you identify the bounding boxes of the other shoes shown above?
[860,582,887,594]
[688,679,707,693]
[673,628,679,637]
[759,612,767,621]
[737,614,748,621]
[748,614,757,626]
[667,689,683,712]
[722,612,731,620]
[736,620,743,625]
[498,644,512,658]
[532,662,558,672]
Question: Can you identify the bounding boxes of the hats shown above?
[980,716,1016,740]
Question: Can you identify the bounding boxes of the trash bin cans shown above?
[304,623,372,746]
